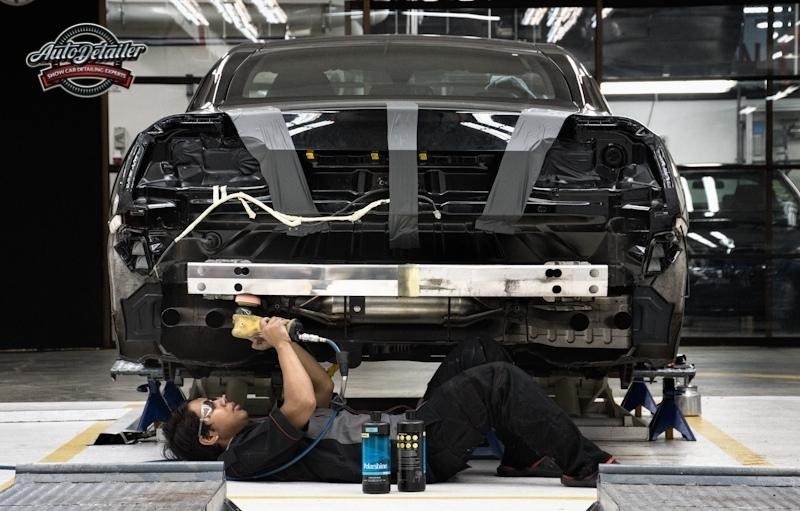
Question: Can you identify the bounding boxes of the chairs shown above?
[264,55,337,98]
[369,57,434,97]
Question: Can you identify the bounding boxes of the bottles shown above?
[676,385,701,417]
[361,409,426,494]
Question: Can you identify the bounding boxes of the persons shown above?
[162,316,622,486]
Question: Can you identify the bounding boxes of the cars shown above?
[671,156,800,335]
[101,31,698,392]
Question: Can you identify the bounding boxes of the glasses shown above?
[198,396,217,437]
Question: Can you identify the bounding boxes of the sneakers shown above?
[496,455,621,487]
